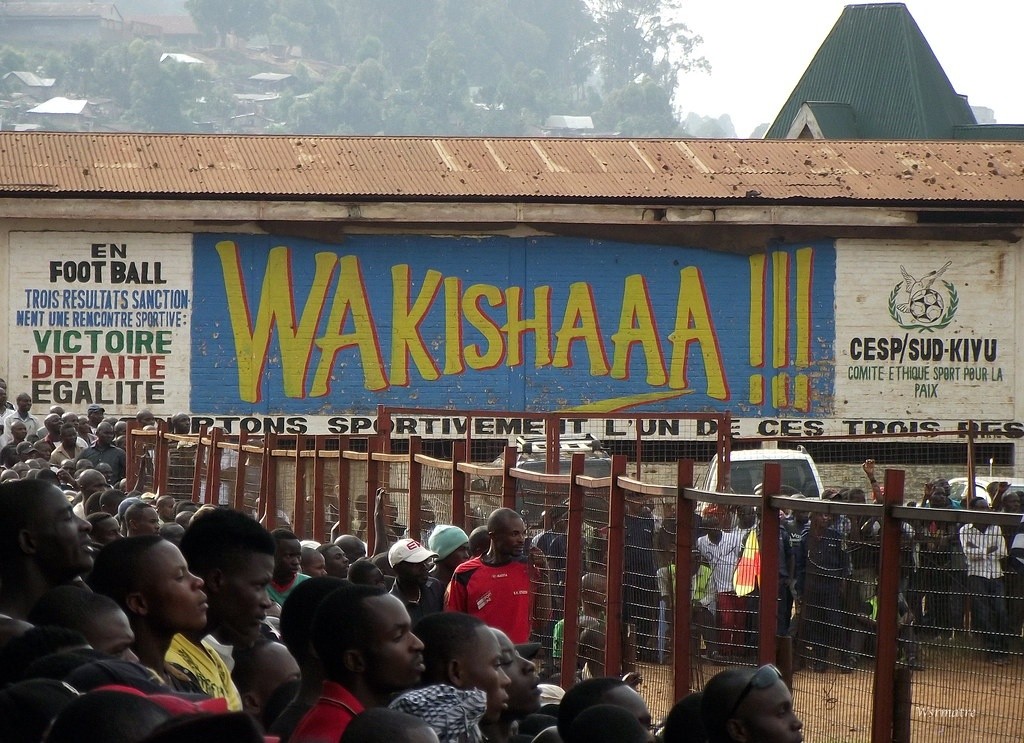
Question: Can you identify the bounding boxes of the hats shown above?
[427,523,470,563]
[15,441,39,455]
[0,647,233,743]
[387,537,439,569]
[88,405,105,413]
[515,642,542,659]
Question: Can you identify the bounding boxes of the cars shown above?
[926,478,1024,512]
[697,444,826,526]
[472,432,628,525]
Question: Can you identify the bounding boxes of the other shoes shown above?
[993,655,1012,666]
[814,658,828,673]
[910,657,926,670]
[842,657,858,674]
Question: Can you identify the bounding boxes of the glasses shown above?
[728,662,784,718]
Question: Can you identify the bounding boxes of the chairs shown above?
[731,469,753,495]
[781,467,801,493]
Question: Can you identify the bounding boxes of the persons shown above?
[223,458,1024,675]
[0,376,413,743]
[385,508,806,743]
